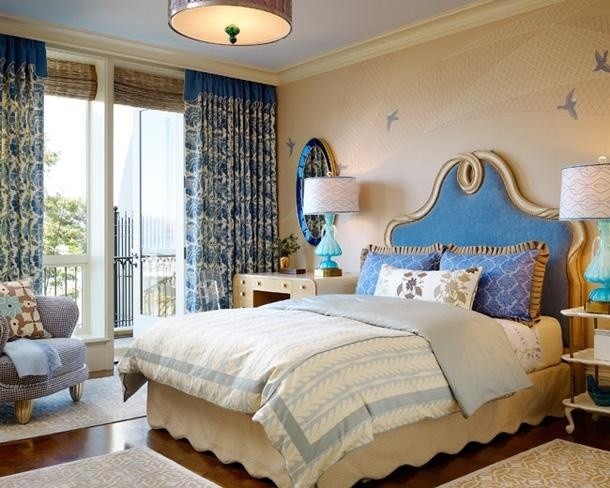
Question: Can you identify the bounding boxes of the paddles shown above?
[436,437,610,487]
[0,445,221,488]
[0,375,147,444]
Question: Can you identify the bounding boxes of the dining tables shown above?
[266,233,300,269]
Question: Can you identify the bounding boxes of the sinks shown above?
[0,284,91,421]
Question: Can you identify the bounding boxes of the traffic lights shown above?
[374,262,482,311]
[355,242,443,296]
[439,240,549,326]
[0,278,53,340]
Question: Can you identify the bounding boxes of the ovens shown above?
[117,146,587,488]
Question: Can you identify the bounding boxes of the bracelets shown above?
[588,385,610,406]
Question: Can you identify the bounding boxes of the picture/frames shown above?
[232,268,358,308]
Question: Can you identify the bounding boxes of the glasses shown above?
[558,155,610,316]
[168,0,292,47]
[304,174,360,276]
